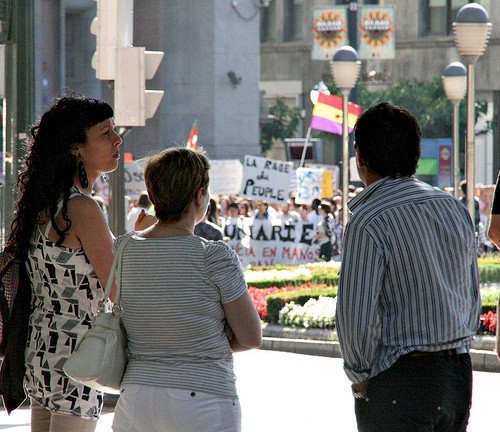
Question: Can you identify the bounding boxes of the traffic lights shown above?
[113,47,165,127]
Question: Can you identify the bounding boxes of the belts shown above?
[407,347,469,356]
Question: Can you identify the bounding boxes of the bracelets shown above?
[351,385,367,398]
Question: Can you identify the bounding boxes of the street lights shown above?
[452,3,493,236]
[329,45,363,240]
[442,60,468,200]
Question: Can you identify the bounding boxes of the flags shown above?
[187,119,198,149]
[309,92,361,136]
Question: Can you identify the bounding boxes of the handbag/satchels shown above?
[0,223,36,361]
[63,233,134,395]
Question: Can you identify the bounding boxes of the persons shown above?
[335,101,482,432]
[458,180,481,259]
[0,93,158,432]
[125,195,156,233]
[110,145,263,432]
[487,171,500,252]
[193,186,364,262]
